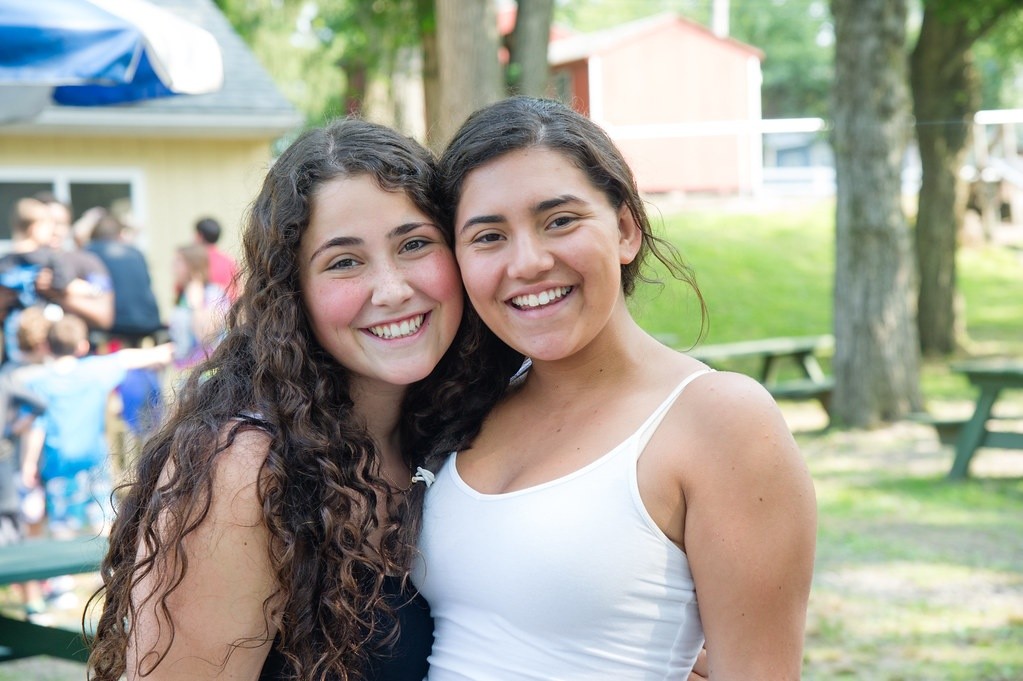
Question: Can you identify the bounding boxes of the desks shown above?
[684,339,849,425]
[0,536,109,681]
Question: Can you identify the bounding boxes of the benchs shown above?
[935,414,1018,449]
[765,378,844,402]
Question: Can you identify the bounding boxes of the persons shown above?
[0,197,167,626]
[87,216,159,349]
[177,220,235,299]
[7,315,174,613]
[410,96,820,677]
[173,250,231,364]
[80,121,492,681]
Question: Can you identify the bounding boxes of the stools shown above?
[933,355,1023,499]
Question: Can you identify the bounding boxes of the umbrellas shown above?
[0,0,220,106]
